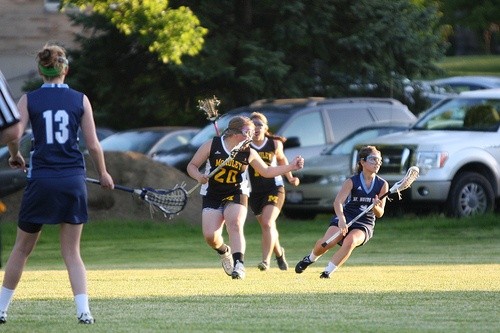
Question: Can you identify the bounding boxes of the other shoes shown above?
[0,310,7,323]
[78,313,95,323]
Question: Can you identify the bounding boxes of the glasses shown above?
[241,126,255,137]
[366,155,382,165]
[252,120,265,129]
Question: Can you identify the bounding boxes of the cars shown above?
[0,126,201,197]
[159,97,419,220]
[351,75,500,217]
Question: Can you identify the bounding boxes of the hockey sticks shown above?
[199,97,221,137]
[188,138,253,195]
[11,160,187,214]
[321,166,420,248]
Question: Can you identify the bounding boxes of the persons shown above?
[0,71,22,148]
[187,116,304,279]
[0,46,114,323]
[295,147,389,278]
[250,113,299,271]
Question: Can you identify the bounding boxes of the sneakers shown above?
[232,259,245,278]
[295,255,314,274]
[220,245,234,276]
[258,261,269,271]
[276,248,288,270]
[319,271,330,278]
